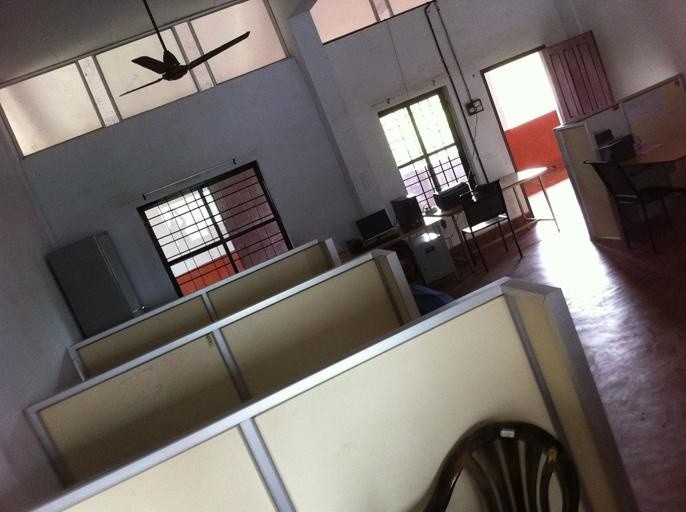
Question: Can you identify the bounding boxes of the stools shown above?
[383,241,428,288]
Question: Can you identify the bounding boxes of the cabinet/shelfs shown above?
[43,231,148,337]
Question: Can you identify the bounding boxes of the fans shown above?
[115,0,252,98]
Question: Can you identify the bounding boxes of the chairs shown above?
[423,419,582,511]
[460,179,525,271]
[583,157,686,253]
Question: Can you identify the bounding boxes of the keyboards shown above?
[368,233,400,249]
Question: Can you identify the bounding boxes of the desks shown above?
[608,141,686,176]
[339,214,460,286]
[425,165,560,274]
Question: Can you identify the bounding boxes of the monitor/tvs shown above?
[356,208,393,240]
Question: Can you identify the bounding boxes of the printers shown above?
[433,182,471,209]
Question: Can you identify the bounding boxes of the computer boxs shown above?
[391,196,425,230]
